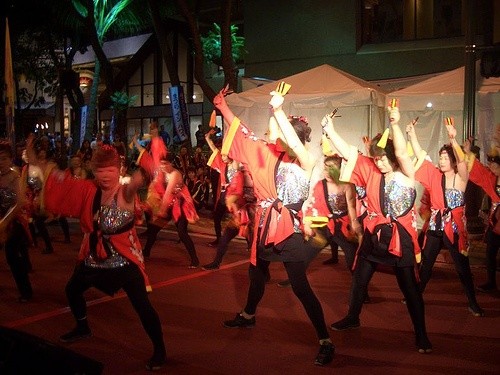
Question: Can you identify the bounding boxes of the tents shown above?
[223,64,500,217]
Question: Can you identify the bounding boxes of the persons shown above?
[213,90,336,365]
[205,130,254,269]
[322,107,432,353]
[209,176,227,246]
[277,155,370,303]
[21,135,60,254]
[61,155,87,182]
[133,137,200,269]
[401,122,484,316]
[0,141,37,303]
[14,122,222,210]
[22,122,167,369]
[464,138,500,292]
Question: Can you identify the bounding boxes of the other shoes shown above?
[279,278,293,288]
[480,283,496,292]
[137,230,148,239]
[469,302,484,317]
[323,256,339,265]
[330,317,360,330]
[208,238,220,247]
[416,336,433,354]
[41,247,54,254]
[224,312,256,328]
[313,342,336,365]
[145,351,166,371]
[60,319,90,342]
[200,262,219,271]
[19,292,33,302]
[188,257,200,269]
